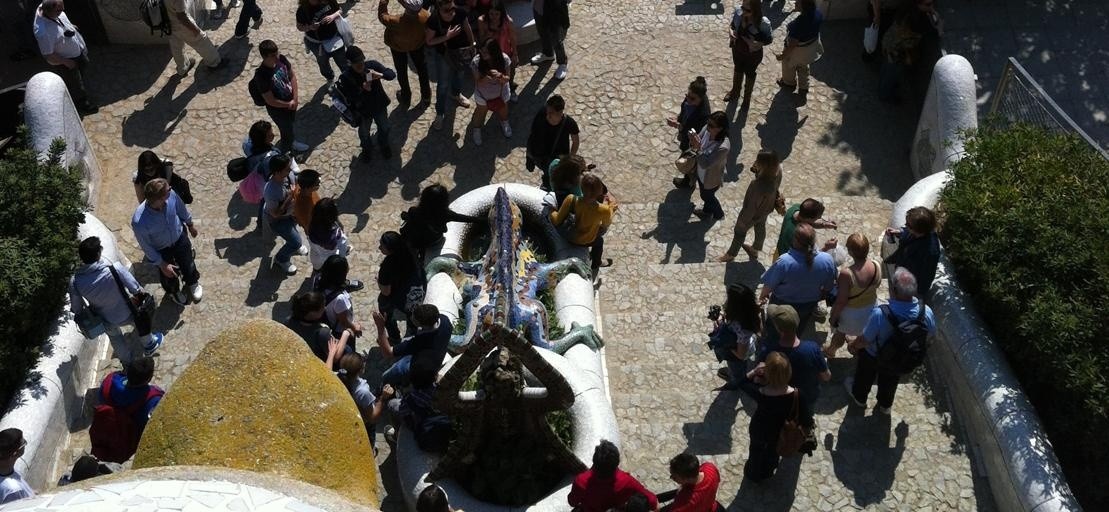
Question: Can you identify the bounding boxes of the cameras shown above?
[689,127,696,137]
[365,70,372,82]
[63,29,74,37]
[138,293,154,312]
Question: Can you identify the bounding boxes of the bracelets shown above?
[344,328,352,337]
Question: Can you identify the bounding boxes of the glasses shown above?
[164,185,171,193]
[13,438,27,456]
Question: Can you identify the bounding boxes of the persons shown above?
[708,287,760,390]
[525,94,593,191]
[477,1,522,104]
[737,351,817,458]
[715,149,782,262]
[213,0,241,18]
[530,0,571,79]
[378,0,431,106]
[757,303,831,429]
[658,453,721,511]
[0,429,38,506]
[324,337,394,459]
[864,0,882,62]
[90,355,164,462]
[132,149,193,204]
[375,231,421,340]
[263,154,308,274]
[723,0,773,112]
[675,112,731,221]
[295,1,352,91]
[470,37,513,146]
[399,184,488,250]
[846,268,938,416]
[426,1,476,130]
[313,255,363,352]
[416,484,462,511]
[884,206,940,304]
[775,0,824,94]
[327,45,396,161]
[131,178,203,306]
[666,76,711,189]
[69,236,164,371]
[235,1,263,39]
[227,120,283,202]
[248,40,309,153]
[545,174,613,268]
[821,232,882,358]
[567,439,657,512]
[57,455,104,486]
[296,169,321,236]
[285,291,354,371]
[876,8,932,106]
[373,304,453,391]
[759,224,837,338]
[33,0,98,114]
[773,198,825,263]
[139,0,230,76]
[307,197,364,292]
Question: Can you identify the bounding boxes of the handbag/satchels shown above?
[239,154,270,203]
[73,280,107,339]
[109,265,155,336]
[557,196,579,240]
[335,14,354,46]
[331,76,360,127]
[527,114,567,171]
[775,387,806,458]
[164,160,193,204]
[227,148,270,181]
[436,9,476,70]
[675,148,699,175]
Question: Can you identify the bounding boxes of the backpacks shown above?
[248,68,272,106]
[877,298,929,375]
[327,74,362,128]
[227,157,249,181]
[313,273,344,337]
[86,374,165,464]
[241,171,266,203]
[139,1,172,38]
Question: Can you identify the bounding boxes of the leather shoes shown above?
[725,87,739,101]
[695,207,710,215]
[776,80,795,89]
[800,88,812,93]
[361,148,370,162]
[672,178,694,189]
[380,138,391,157]
[215,4,224,18]
[742,94,752,111]
[712,213,724,220]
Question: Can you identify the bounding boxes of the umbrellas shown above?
[709,305,722,326]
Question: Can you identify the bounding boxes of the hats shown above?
[346,46,365,62]
[767,304,800,331]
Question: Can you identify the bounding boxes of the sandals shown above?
[594,258,613,267]
[744,241,757,258]
[710,254,734,262]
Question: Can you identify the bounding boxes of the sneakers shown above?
[170,290,187,305]
[472,125,482,146]
[845,377,867,406]
[555,63,568,79]
[345,280,363,290]
[183,58,195,74]
[208,55,230,70]
[80,101,98,113]
[145,332,163,358]
[501,119,512,137]
[254,15,260,23]
[275,256,297,274]
[123,362,130,373]
[294,244,308,256]
[189,281,203,302]
[878,398,896,414]
[231,0,240,7]
[234,28,250,38]
[384,425,397,444]
[451,92,470,107]
[434,113,445,128]
[292,141,311,152]
[531,53,554,64]
